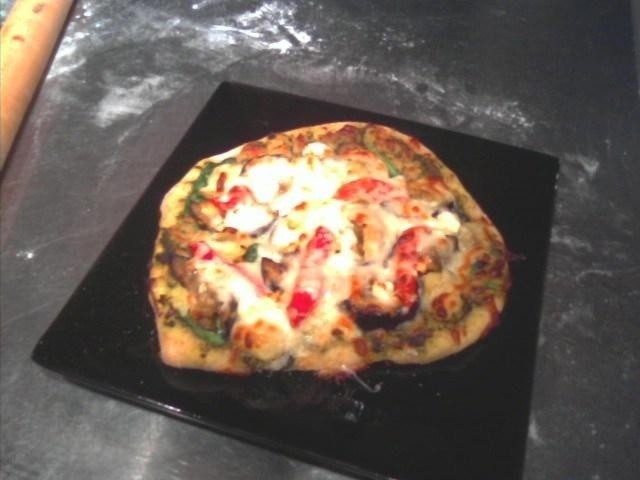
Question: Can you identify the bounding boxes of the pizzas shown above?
[150,122,508,378]
